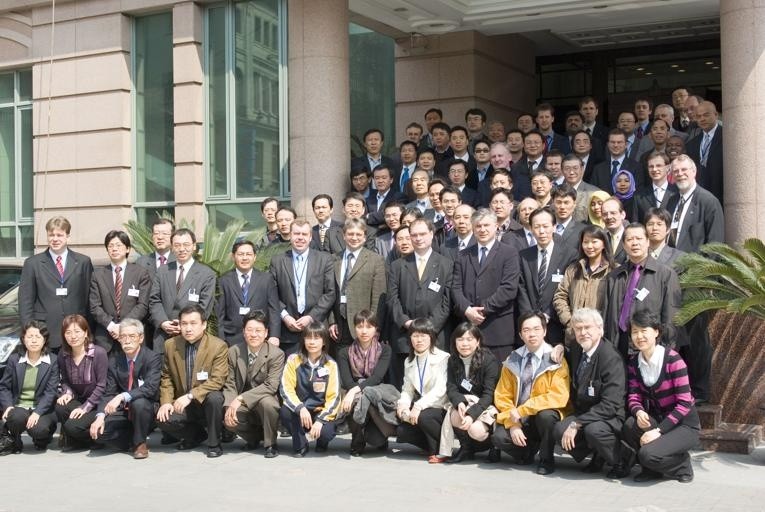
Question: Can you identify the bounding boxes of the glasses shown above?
[473,148,490,154]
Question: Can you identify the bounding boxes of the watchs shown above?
[188,392,193,400]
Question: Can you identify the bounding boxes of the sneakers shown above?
[0,441,24,457]
[61,444,79,453]
[427,453,450,465]
[32,443,48,454]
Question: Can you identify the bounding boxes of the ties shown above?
[399,165,410,196]
[113,265,124,318]
[519,353,535,423]
[55,255,65,281]
[667,195,686,250]
[339,252,355,321]
[295,253,307,314]
[458,240,465,252]
[655,186,664,209]
[479,245,489,272]
[537,248,549,311]
[682,118,689,130]
[554,223,567,237]
[610,235,617,258]
[611,158,620,180]
[436,213,444,225]
[528,160,537,178]
[698,133,711,169]
[636,124,644,140]
[616,262,641,333]
[575,352,589,387]
[156,254,167,266]
[127,360,135,393]
[416,256,426,283]
[528,231,538,249]
[586,128,592,135]
[319,224,326,247]
[175,265,186,293]
[239,273,250,306]
[247,353,257,381]
[445,221,454,237]
[623,140,630,160]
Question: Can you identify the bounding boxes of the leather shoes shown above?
[606,448,638,479]
[159,431,183,446]
[262,444,280,459]
[678,473,694,483]
[293,441,310,458]
[314,439,330,453]
[515,445,539,467]
[483,448,503,464]
[349,445,365,458]
[535,460,556,475]
[133,442,150,460]
[633,469,664,482]
[443,446,474,463]
[375,440,389,456]
[220,431,237,443]
[395,435,408,444]
[280,430,293,437]
[239,439,261,452]
[581,455,605,473]
[205,444,224,458]
[335,426,350,435]
[175,429,209,451]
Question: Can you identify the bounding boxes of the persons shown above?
[18,215,96,353]
[222,311,285,458]
[258,85,726,483]
[54,314,108,451]
[135,219,174,275]
[215,241,281,348]
[89,230,151,352]
[82,318,161,459]
[156,304,229,457]
[148,228,217,357]
[0,318,60,454]
